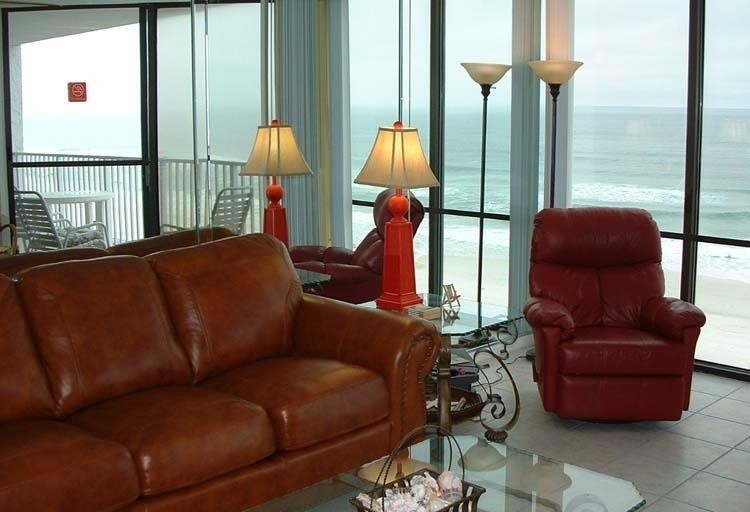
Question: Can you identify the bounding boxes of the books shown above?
[402,304,440,317]
[423,313,441,320]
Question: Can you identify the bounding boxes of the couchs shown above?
[0,233,442,512]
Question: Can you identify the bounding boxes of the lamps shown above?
[236,118,316,248]
[526,57,584,359]
[354,118,441,312]
[459,62,515,346]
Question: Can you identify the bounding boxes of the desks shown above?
[351,293,530,434]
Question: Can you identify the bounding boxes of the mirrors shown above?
[5,0,542,353]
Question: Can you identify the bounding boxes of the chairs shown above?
[159,187,255,241]
[524,206,707,425]
[287,186,424,304]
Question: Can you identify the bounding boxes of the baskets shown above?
[350,424,486,512]
